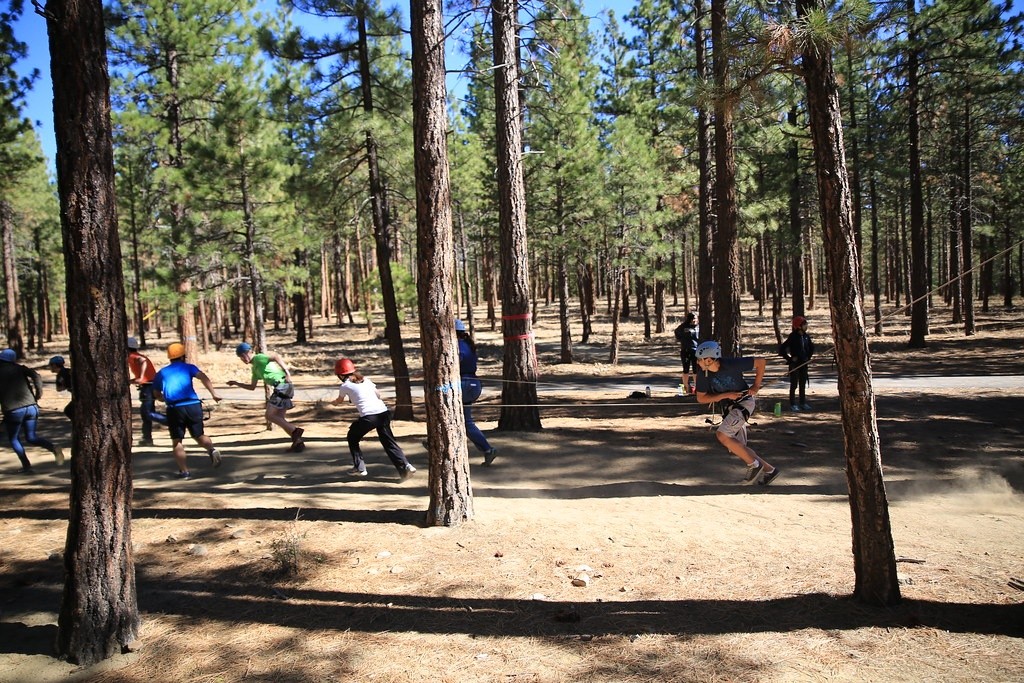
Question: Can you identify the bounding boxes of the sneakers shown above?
[740,461,764,485]
[756,467,782,486]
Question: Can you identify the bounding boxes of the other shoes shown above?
[209,448,222,467]
[346,467,367,478]
[800,403,812,412]
[16,467,34,475]
[480,447,498,466]
[789,405,800,412]
[290,427,304,443]
[173,470,192,481]
[139,438,154,447]
[285,443,305,453]
[399,464,417,483]
[421,441,429,450]
[51,445,65,466]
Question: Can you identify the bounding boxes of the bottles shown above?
[646,387,650,397]
[775,403,781,417]
[678,381,695,396]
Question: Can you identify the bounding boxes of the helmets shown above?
[0,349,16,363]
[128,336,139,349]
[454,319,467,330]
[333,357,357,374]
[792,315,806,331]
[167,341,186,360]
[235,343,252,357]
[694,341,723,359]
[49,356,65,365]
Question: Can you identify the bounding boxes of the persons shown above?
[675,313,698,392]
[695,341,781,486]
[152,343,222,479]
[49,356,72,421]
[332,358,416,480]
[0,349,64,473]
[226,343,305,450]
[780,317,814,411]
[128,337,168,445]
[421,321,499,468]
